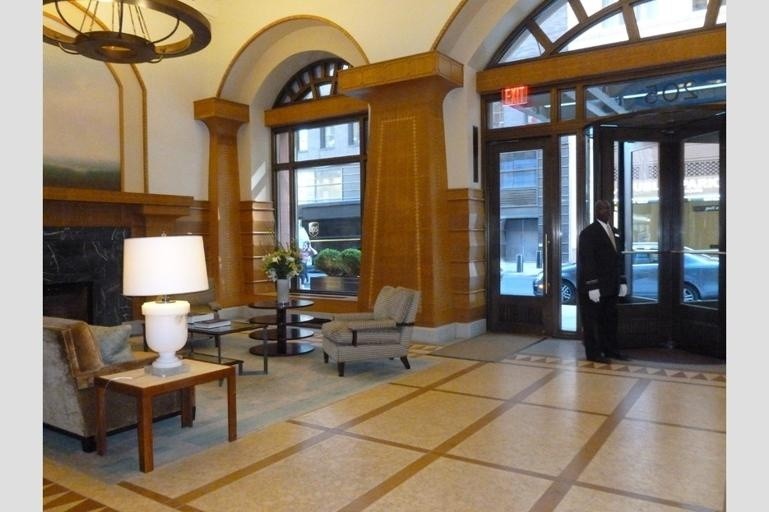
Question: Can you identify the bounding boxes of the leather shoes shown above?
[587,347,629,362]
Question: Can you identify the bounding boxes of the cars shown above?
[532,240,720,306]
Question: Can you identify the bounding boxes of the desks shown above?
[91,356,240,476]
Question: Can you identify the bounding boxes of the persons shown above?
[575,198,629,366]
[298,240,318,284]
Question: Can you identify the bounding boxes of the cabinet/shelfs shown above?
[247,299,316,358]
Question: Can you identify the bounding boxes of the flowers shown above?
[259,222,312,284]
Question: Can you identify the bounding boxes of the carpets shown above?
[42,328,443,488]
[425,330,548,363]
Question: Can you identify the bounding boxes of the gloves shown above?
[589,288,601,303]
[618,283,628,298]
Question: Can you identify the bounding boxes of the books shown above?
[193,318,232,329]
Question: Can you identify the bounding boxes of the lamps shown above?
[121,233,211,369]
[42,0,214,66]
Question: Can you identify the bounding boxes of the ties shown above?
[607,226,616,249]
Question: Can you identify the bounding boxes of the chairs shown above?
[44,314,199,454]
[321,286,422,381]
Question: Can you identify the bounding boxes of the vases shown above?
[276,279,291,305]
